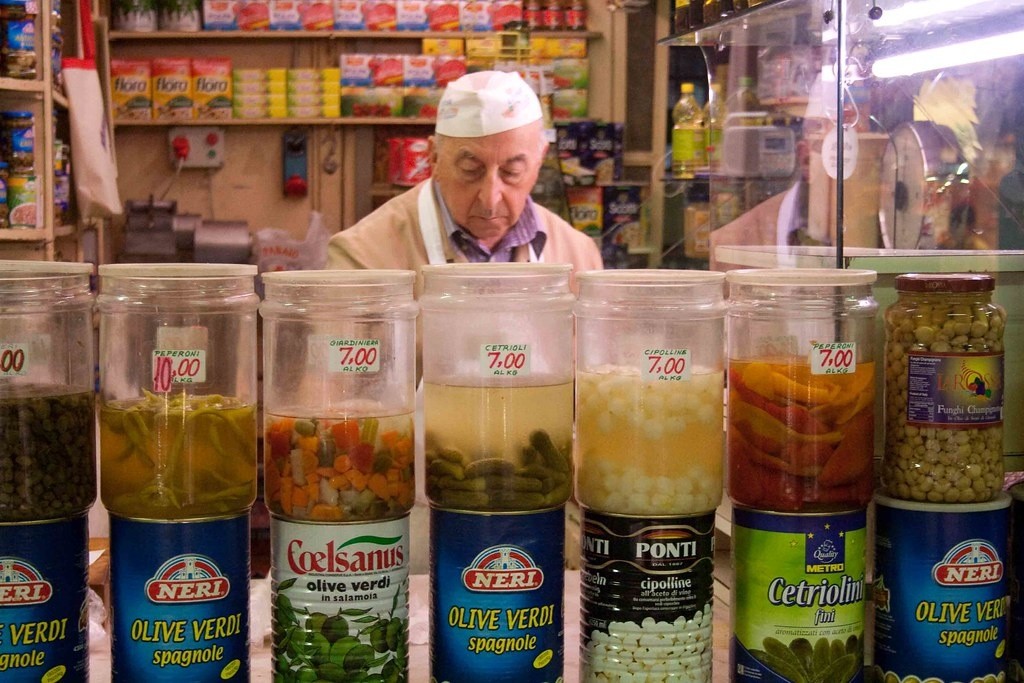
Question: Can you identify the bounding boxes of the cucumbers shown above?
[424,429,573,510]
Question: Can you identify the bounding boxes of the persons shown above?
[321,69,602,426]
[712,66,841,280]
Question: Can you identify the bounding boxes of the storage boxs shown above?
[341,88,403,117]
[602,186,643,250]
[404,55,467,86]
[466,39,499,57]
[288,68,341,118]
[530,59,590,90]
[403,87,447,117]
[340,54,404,87]
[422,38,465,56]
[531,38,586,58]
[553,122,596,187]
[577,121,624,183]
[562,188,602,249]
[233,68,288,118]
[537,88,587,117]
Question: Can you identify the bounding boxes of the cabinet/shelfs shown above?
[0,0,109,263]
[107,0,603,125]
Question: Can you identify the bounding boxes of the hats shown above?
[433,71,544,138]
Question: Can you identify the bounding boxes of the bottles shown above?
[728,77,763,127]
[671,82,707,180]
[703,84,728,172]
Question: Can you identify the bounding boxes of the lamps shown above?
[858,25,1024,79]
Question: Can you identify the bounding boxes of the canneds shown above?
[523,0,587,31]
[0,0,63,91]
[0,107,70,233]
